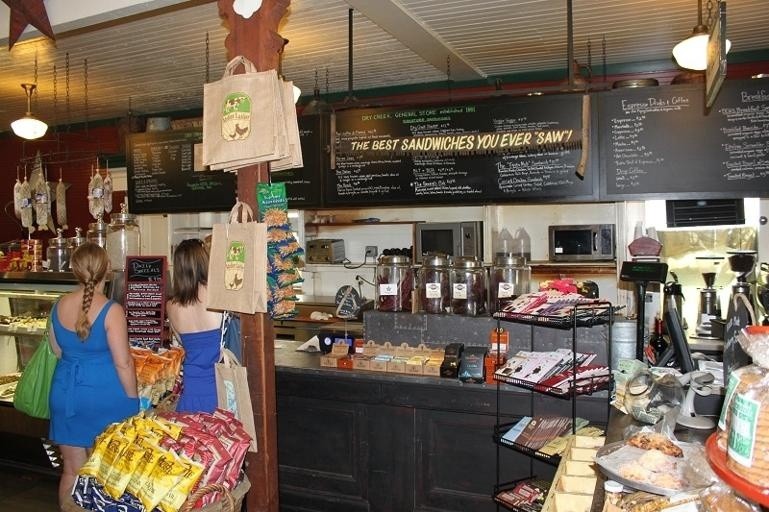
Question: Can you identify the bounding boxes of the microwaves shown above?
[548,224,616,262]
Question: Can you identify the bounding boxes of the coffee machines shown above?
[695,249,757,340]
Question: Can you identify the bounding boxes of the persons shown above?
[47,241,141,512]
[165,237,239,416]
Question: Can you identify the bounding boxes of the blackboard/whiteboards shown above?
[324,86,599,209]
[124,256,167,348]
[125,110,324,214]
[598,79,769,202]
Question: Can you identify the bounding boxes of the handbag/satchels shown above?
[201,56,304,175]
[227,316,243,363]
[214,361,258,456]
[206,203,267,313]
[14,332,59,420]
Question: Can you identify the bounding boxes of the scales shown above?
[619,262,667,360]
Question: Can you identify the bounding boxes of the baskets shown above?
[186,467,253,512]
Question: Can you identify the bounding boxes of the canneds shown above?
[14,238,44,272]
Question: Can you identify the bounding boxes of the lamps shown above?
[11,84,49,141]
[673,0,731,71]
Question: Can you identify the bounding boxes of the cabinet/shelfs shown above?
[1,270,113,440]
[493,297,612,510]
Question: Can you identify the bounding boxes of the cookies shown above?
[720,374,769,486]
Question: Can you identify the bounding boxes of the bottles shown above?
[496,227,530,263]
[648,318,668,368]
[663,271,683,328]
[376,255,532,316]
[0,203,140,272]
[625,369,686,424]
[605,480,623,505]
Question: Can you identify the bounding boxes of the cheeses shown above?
[551,435,606,512]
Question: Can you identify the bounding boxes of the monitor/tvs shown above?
[415,223,461,264]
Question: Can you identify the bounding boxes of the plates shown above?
[336,286,361,320]
